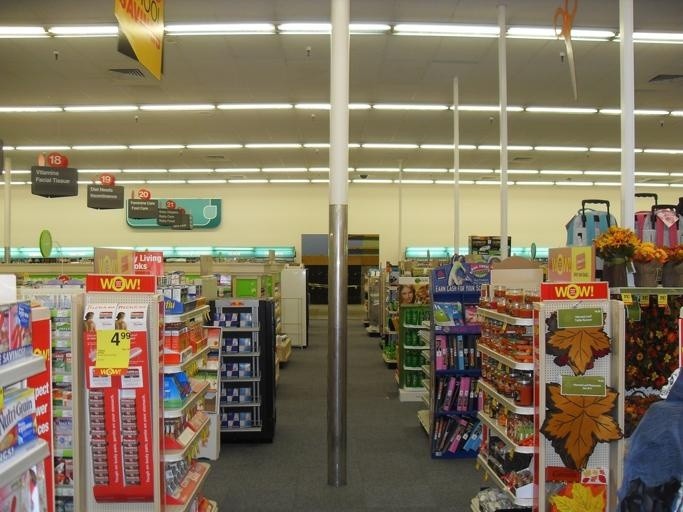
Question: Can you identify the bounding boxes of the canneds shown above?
[479,284,540,407]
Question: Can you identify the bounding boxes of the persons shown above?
[114,311,128,331]
[447,254,473,286]
[399,285,415,305]
[83,312,97,334]
